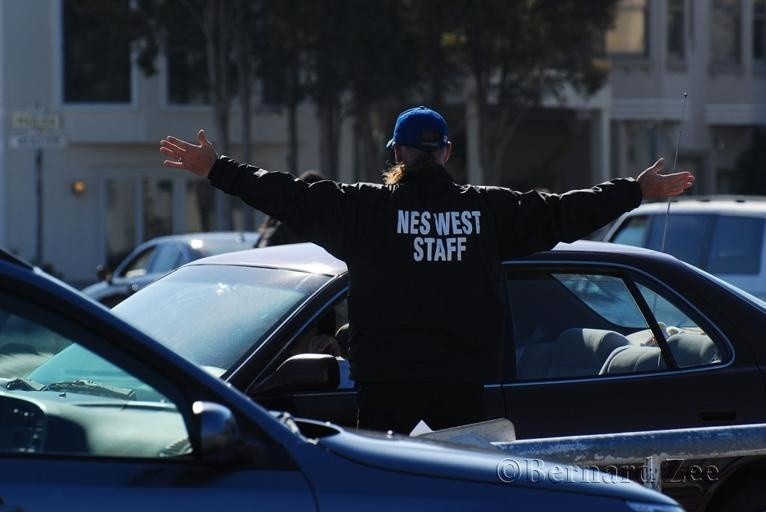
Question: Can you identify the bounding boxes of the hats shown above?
[385,105,451,152]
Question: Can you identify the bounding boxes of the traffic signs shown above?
[9,111,63,129]
[6,131,69,150]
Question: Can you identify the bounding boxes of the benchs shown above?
[516,323,718,377]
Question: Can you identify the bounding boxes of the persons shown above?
[283,321,340,359]
[158,107,696,431]
[261,168,326,246]
